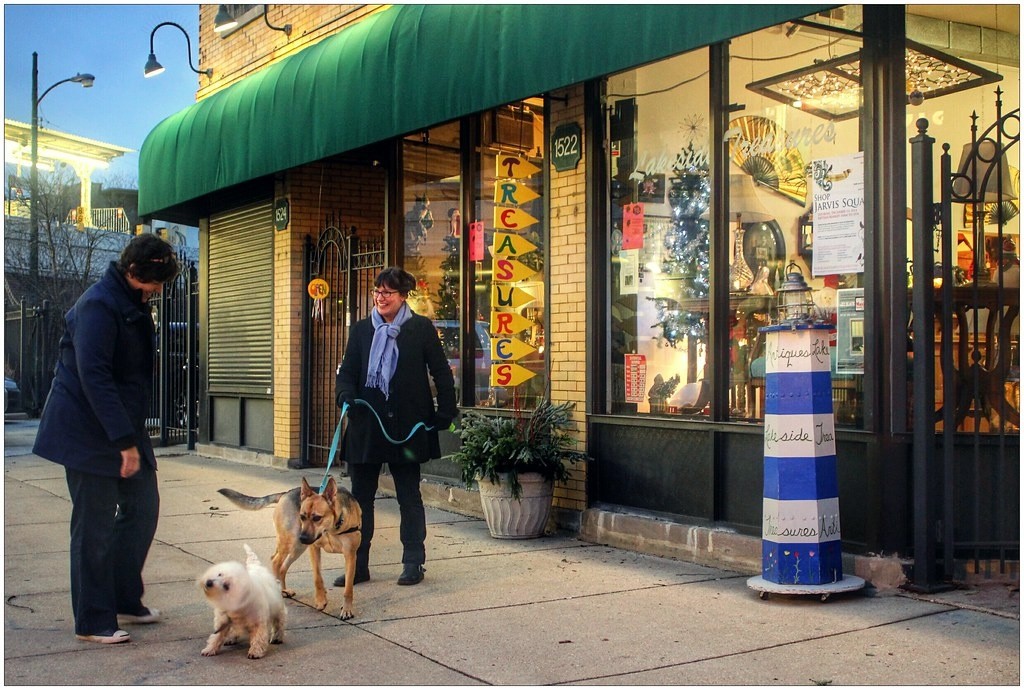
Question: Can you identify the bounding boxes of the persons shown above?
[33,234,175,643]
[334,266,457,588]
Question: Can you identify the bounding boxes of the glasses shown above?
[148,251,178,264]
[370,289,399,298]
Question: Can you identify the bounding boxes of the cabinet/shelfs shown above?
[908,332,997,432]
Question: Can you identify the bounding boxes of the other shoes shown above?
[335,568,370,586]
[76,627,130,642]
[117,607,161,625]
[397,561,424,584]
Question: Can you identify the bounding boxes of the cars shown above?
[146,321,202,428]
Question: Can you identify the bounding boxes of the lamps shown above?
[951,142,1018,286]
[797,205,812,256]
[729,174,777,293]
[144,22,213,79]
[776,263,817,325]
[213,4,292,36]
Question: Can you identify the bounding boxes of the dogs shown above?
[198,542,285,658]
[217,476,362,622]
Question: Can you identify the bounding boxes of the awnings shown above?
[138,4,836,225]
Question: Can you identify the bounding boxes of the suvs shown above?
[432,320,508,405]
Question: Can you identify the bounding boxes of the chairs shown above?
[747,329,856,423]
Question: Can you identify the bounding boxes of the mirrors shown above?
[733,219,787,290]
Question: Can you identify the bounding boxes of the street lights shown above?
[29,73,95,284]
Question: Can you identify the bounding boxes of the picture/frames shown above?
[639,215,710,280]
[637,170,666,204]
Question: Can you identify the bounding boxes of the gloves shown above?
[338,391,356,409]
[432,411,453,430]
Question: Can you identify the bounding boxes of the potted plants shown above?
[440,400,595,541]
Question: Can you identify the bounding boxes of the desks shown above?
[906,286,1020,429]
[679,293,777,417]
